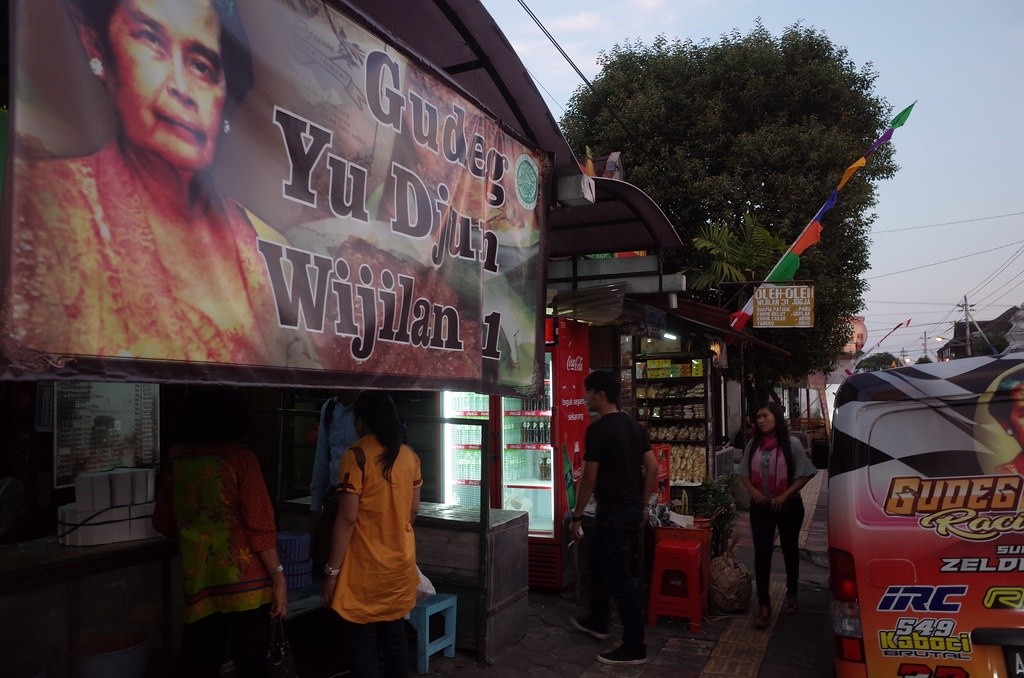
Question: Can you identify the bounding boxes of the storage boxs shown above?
[56,466,164,546]
[655,517,711,591]
[282,564,314,589]
[650,441,671,509]
[275,530,311,562]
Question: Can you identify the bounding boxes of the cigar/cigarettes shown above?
[568,540,575,547]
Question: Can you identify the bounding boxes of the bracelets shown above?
[270,564,287,573]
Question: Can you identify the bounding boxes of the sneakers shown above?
[573,616,612,638]
[596,643,649,665]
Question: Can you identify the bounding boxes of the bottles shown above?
[521,384,551,481]
[562,442,583,512]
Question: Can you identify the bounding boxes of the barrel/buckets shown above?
[46,628,154,678]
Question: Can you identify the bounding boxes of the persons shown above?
[568,368,658,668]
[739,401,819,630]
[150,385,292,678]
[310,388,422,677]
[5,1,322,369]
[987,366,1024,479]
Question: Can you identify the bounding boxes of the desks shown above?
[283,493,530,665]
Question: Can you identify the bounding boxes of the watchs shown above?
[569,512,582,521]
[326,563,342,580]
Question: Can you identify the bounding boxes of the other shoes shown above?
[783,592,799,613]
[754,597,773,619]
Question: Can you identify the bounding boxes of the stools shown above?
[648,539,704,635]
[406,594,457,673]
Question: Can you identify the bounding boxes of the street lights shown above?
[936,337,971,355]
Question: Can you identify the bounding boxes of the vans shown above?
[828,349,1024,678]
